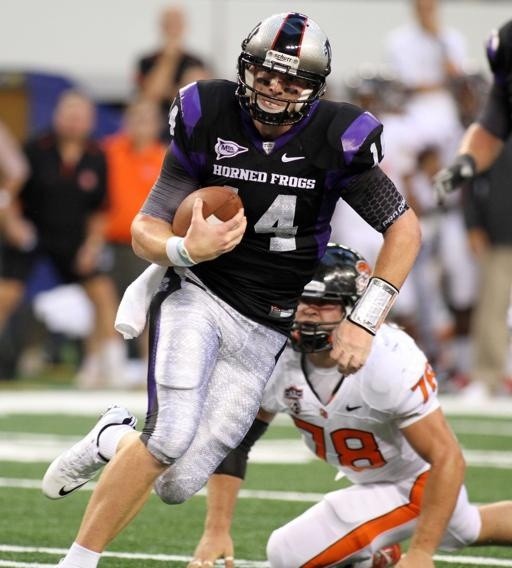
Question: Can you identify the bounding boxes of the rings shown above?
[191,561,202,566]
[203,561,213,567]
[224,557,233,561]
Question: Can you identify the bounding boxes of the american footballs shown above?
[171,187,242,236]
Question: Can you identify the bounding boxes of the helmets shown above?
[287,243,373,353]
[234,13,332,127]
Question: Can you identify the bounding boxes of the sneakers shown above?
[373,544,401,567]
[42,405,138,500]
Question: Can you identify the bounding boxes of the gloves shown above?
[433,154,477,213]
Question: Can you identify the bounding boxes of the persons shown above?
[187,244,512,568]
[41,13,424,568]
[0,0,512,389]
